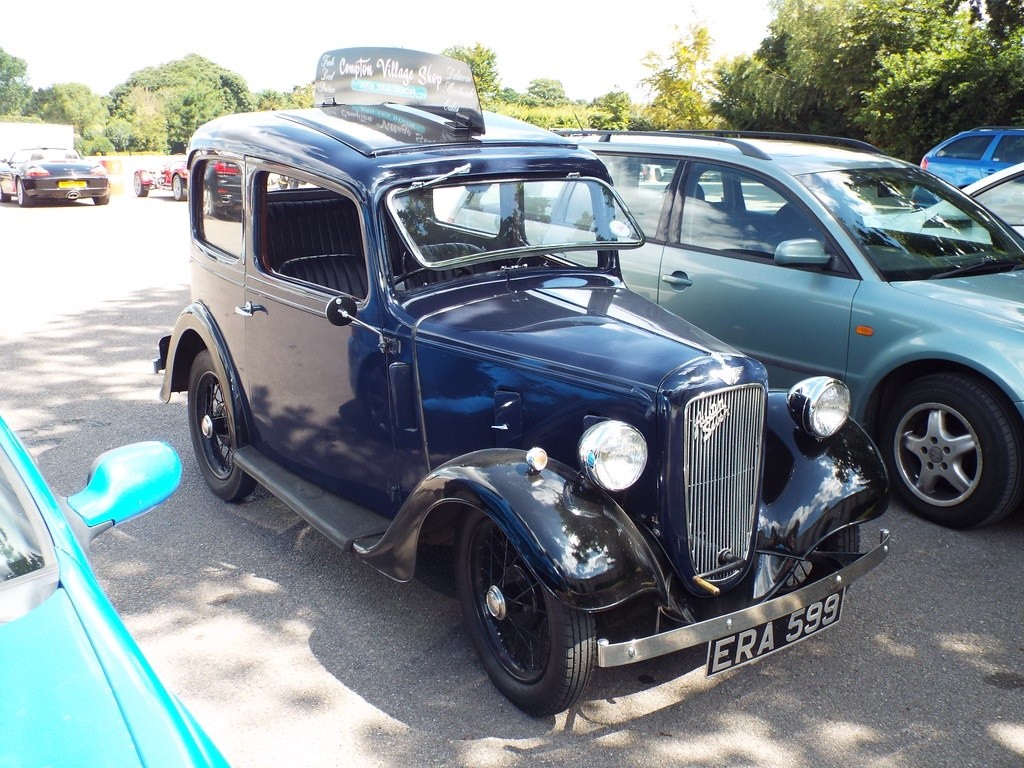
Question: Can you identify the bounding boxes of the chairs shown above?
[403,243,491,290]
[280,254,368,300]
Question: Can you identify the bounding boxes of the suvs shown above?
[911,126,1024,210]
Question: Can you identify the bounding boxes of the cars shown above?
[853,161,1024,244]
[132,158,189,201]
[153,44,897,721]
[0,146,112,207]
[437,127,1024,531]
[196,154,244,218]
[0,411,232,768]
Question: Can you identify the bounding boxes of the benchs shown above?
[214,197,424,275]
